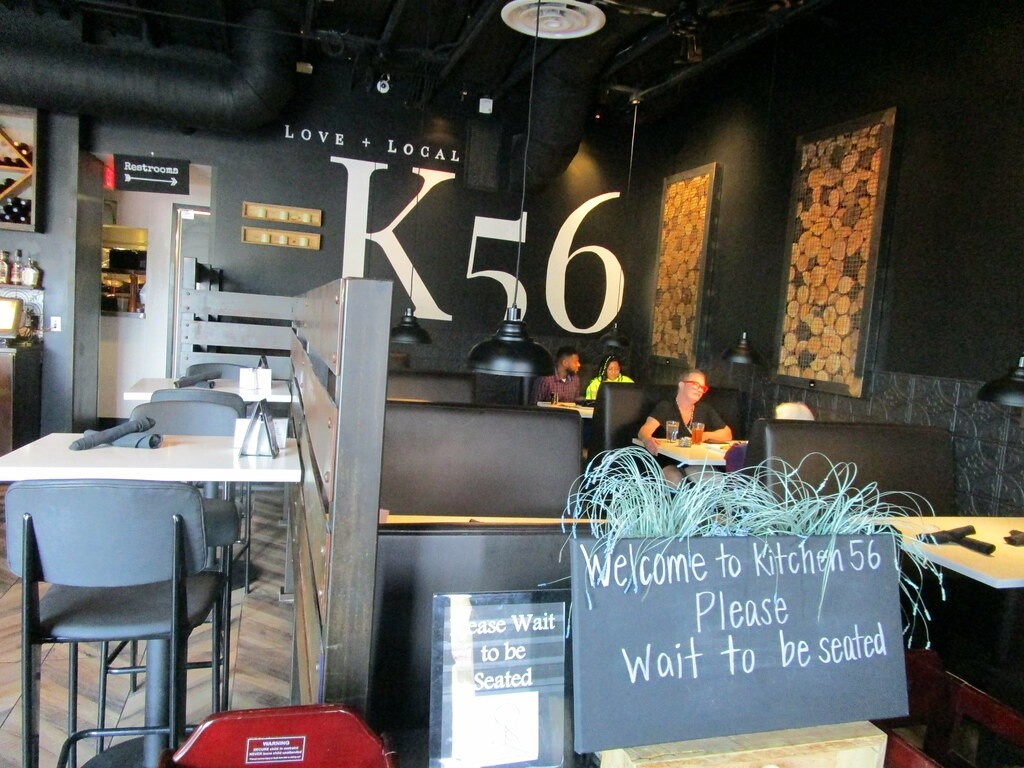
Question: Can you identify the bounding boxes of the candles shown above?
[256,208,267,218]
[298,237,309,246]
[278,235,288,245]
[303,214,312,222]
[261,233,270,242]
[280,211,289,220]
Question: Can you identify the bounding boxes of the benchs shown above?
[744,418,997,652]
[585,382,743,489]
[385,369,475,403]
[943,665,1024,768]
[101,223,148,294]
[576,365,632,397]
[379,400,583,519]
[366,523,623,768]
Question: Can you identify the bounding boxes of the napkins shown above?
[84,429,163,449]
[181,376,216,389]
[69,416,155,451]
[173,370,223,388]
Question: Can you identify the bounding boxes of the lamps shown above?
[975,347,1024,408]
[464,0,558,377]
[599,95,641,347]
[720,25,782,365]
[388,60,432,344]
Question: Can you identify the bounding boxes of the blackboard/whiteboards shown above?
[570,534,910,754]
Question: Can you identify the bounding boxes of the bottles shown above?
[1,249,39,287]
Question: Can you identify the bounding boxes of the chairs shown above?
[3,478,226,768]
[151,389,251,596]
[185,362,251,517]
[94,401,239,756]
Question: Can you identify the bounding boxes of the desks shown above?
[122,378,291,591]
[100,268,146,313]
[856,515,1024,768]
[632,438,750,465]
[0,432,302,768]
[384,513,611,524]
[537,402,594,419]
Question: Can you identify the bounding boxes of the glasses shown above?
[683,381,708,393]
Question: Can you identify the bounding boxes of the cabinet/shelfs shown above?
[0,104,44,233]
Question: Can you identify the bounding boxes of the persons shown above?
[724,401,815,477]
[639,369,732,493]
[536,347,581,403]
[585,355,635,403]
[19,311,31,338]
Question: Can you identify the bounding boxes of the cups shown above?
[666,421,679,443]
[117,298,128,312]
[0,197,32,224]
[691,422,705,445]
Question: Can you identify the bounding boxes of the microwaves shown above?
[109,249,147,272]
